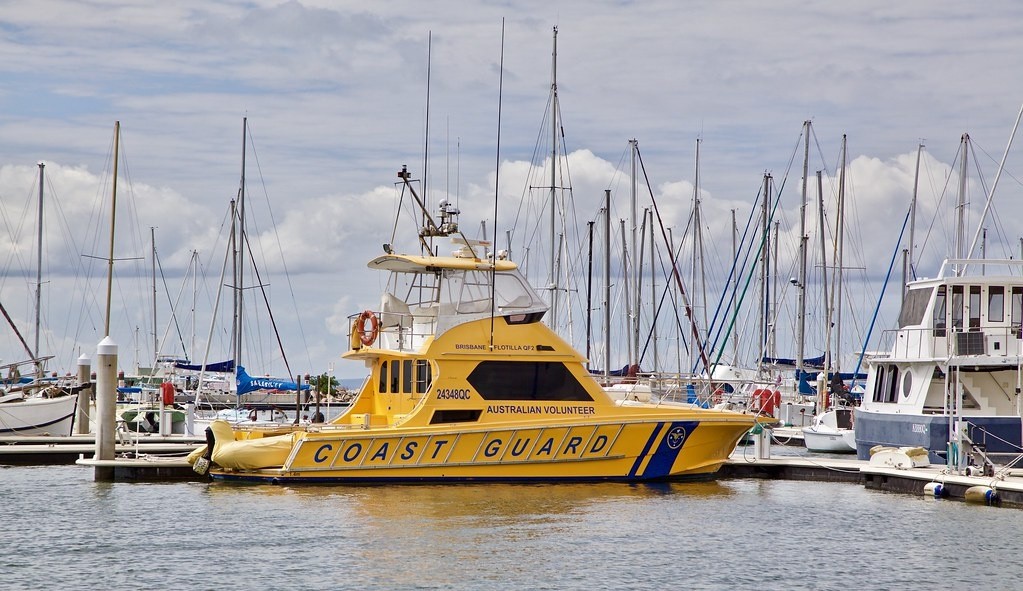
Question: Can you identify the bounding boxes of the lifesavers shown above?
[356,309,379,347]
[713,390,724,402]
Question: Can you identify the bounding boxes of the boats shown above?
[855,257,1023,467]
[188,168,776,486]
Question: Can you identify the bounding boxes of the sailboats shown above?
[1,15,857,454]
